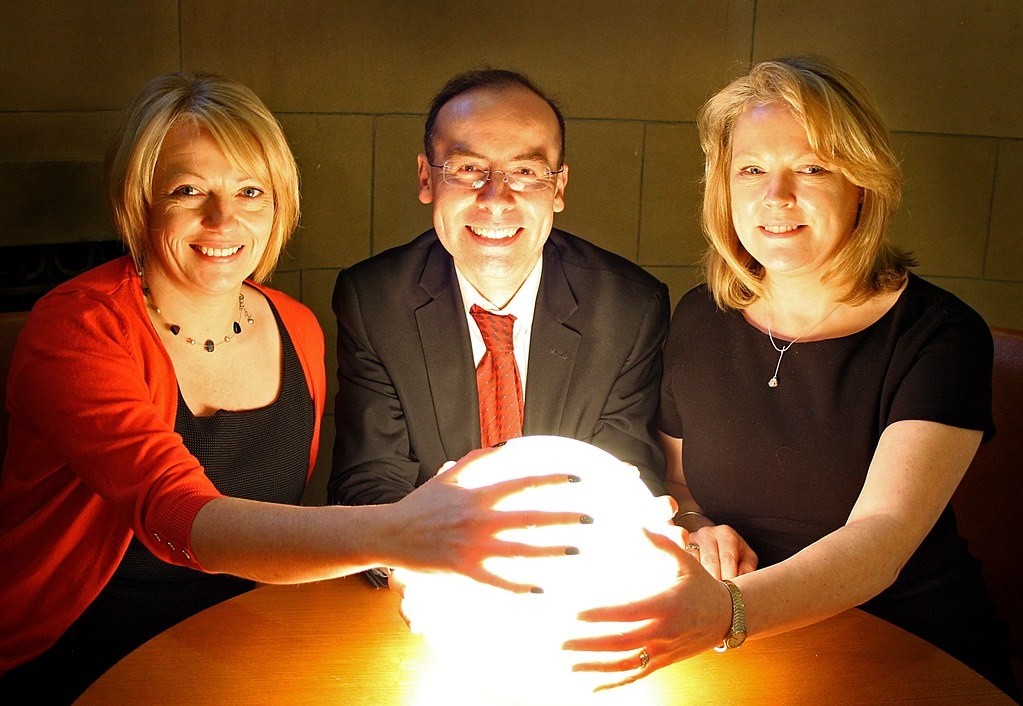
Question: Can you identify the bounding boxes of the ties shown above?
[468,303,524,450]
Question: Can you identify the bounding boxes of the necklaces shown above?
[137,259,254,351]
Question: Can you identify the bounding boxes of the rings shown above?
[762,289,843,387]
[639,648,649,668]
[685,542,699,550]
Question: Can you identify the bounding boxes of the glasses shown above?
[430,159,565,193]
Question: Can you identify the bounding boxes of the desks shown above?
[68,565,1023,706]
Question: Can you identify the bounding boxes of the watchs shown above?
[714,579,750,653]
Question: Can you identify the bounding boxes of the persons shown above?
[562,52,1018,700]
[328,68,690,628]
[0,72,594,706]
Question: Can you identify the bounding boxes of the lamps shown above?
[389,435,689,705]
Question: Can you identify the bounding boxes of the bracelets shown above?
[673,511,704,521]
